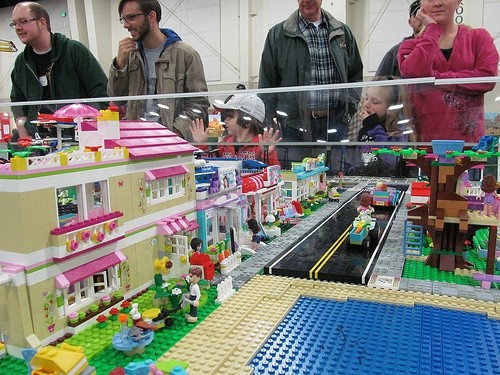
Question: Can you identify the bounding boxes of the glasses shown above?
[119,13,146,24]
[10,17,39,29]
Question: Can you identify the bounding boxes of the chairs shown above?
[22,349,38,375]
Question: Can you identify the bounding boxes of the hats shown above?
[409,0,421,17]
[212,93,265,124]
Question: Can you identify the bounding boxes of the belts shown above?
[308,110,335,118]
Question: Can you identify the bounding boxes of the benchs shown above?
[472,272,500,289]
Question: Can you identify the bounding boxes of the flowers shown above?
[205,244,226,255]
[96,315,108,323]
[68,311,79,320]
[109,308,119,316]
[121,300,131,308]
[154,256,174,275]
[101,293,111,301]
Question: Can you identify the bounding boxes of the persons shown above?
[106,0,211,141]
[258,0,363,142]
[189,92,282,170]
[375,0,423,78]
[355,77,409,176]
[10,2,110,137]
[221,84,246,122]
[396,0,499,142]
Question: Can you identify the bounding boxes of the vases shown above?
[120,307,132,314]
[109,314,118,322]
[96,321,109,329]
[68,317,80,324]
[114,291,123,300]
[90,304,99,313]
[154,273,163,287]
[78,310,87,320]
[206,250,226,262]
[102,300,111,306]
